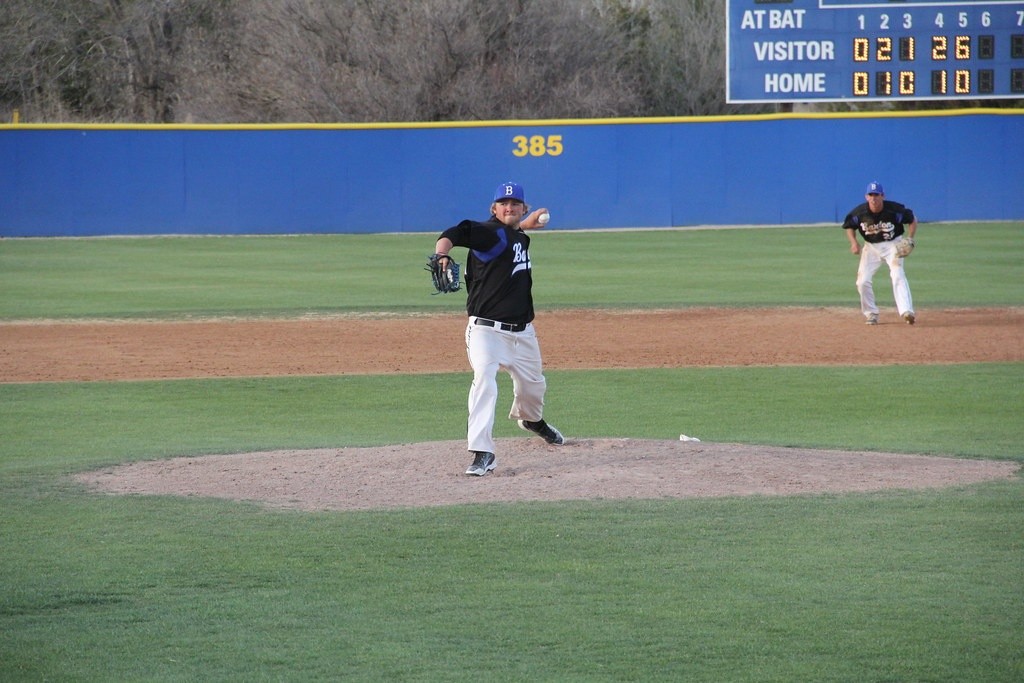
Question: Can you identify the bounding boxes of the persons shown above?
[841,182,917,324]
[428,181,565,476]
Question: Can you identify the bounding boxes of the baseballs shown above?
[538,213,550,224]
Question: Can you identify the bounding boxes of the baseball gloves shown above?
[423,253,466,296]
[894,237,915,258]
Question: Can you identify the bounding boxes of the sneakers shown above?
[466,451,497,476]
[517,419,565,445]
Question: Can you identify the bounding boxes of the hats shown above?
[494,182,524,203]
[866,180,884,194]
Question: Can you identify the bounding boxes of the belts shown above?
[475,319,526,332]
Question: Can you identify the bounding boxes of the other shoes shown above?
[865,318,877,325]
[903,312,915,325]
